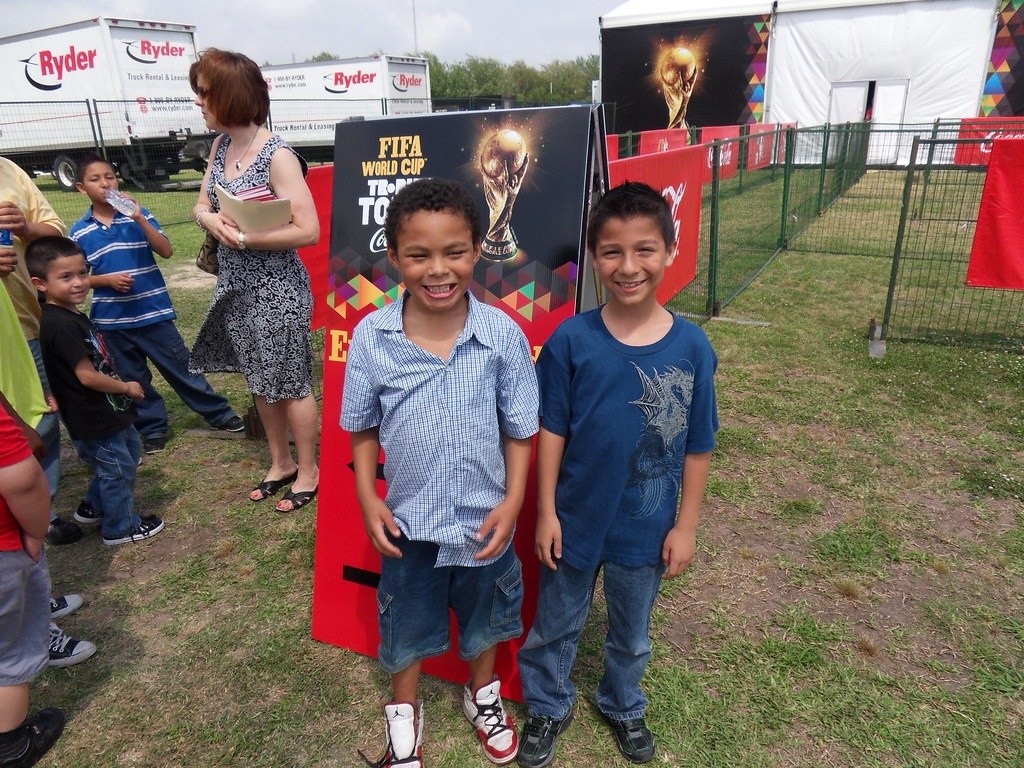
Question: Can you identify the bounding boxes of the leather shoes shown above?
[0,706,64,768]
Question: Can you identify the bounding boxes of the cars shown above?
[183,138,213,159]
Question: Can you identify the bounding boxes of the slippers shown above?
[250,467,299,502]
[276,484,319,512]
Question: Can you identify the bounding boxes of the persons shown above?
[24,236,165,546]
[515,182,720,768]
[0,157,98,768]
[187,47,321,513]
[68,156,245,454]
[340,176,540,768]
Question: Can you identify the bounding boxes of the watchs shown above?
[237,232,247,250]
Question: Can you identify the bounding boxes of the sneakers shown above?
[600,709,655,763]
[210,416,246,432]
[357,698,425,767]
[462,673,518,763]
[102,515,164,545]
[42,618,97,668]
[50,594,83,617]
[74,500,104,523]
[144,437,164,453]
[516,708,573,768]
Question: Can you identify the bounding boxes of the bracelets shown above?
[196,208,208,232]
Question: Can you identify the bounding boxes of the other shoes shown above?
[44,518,82,546]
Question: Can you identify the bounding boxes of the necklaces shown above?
[231,125,259,170]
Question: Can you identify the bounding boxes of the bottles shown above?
[105,188,136,217]
[0,221,15,268]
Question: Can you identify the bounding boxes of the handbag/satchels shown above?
[196,232,221,278]
